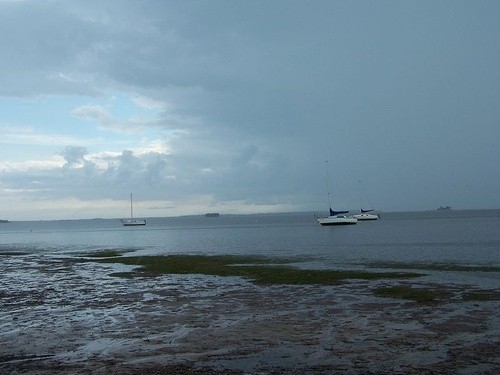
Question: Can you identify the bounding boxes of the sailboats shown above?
[122,192,146,226]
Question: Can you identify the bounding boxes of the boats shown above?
[353,208,380,220]
[437,206,451,210]
[206,213,220,217]
[0,219,8,223]
[317,207,358,225]
